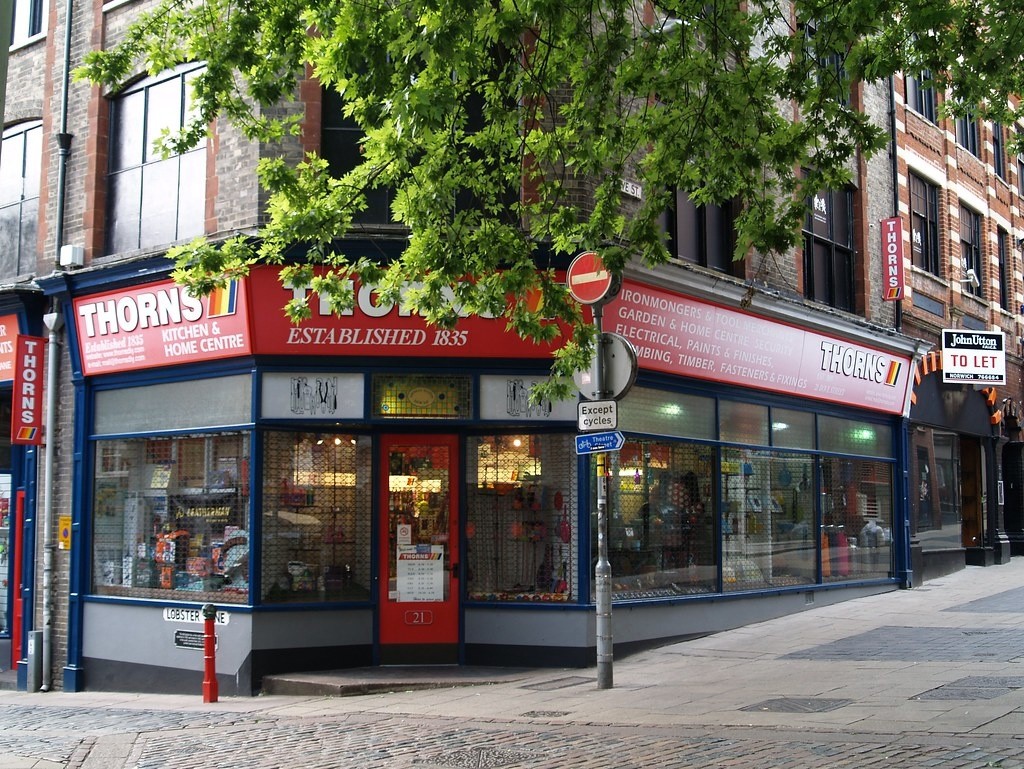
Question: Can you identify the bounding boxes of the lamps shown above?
[314,433,324,445]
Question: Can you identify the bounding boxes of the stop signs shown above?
[566,250,612,304]
